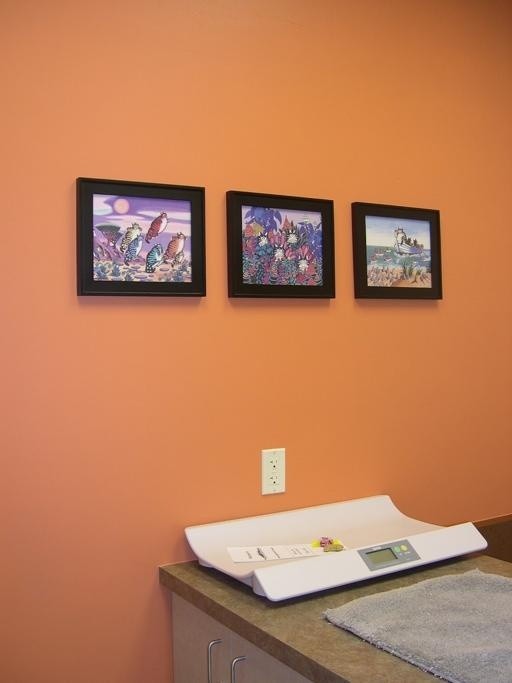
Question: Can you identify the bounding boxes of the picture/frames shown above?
[351,202,443,300]
[226,190,336,299]
[76,177,207,297]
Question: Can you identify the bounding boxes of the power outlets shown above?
[260,448,286,495]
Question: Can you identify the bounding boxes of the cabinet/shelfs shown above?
[171,574,319,682]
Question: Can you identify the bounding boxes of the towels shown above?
[321,568,512,683]
